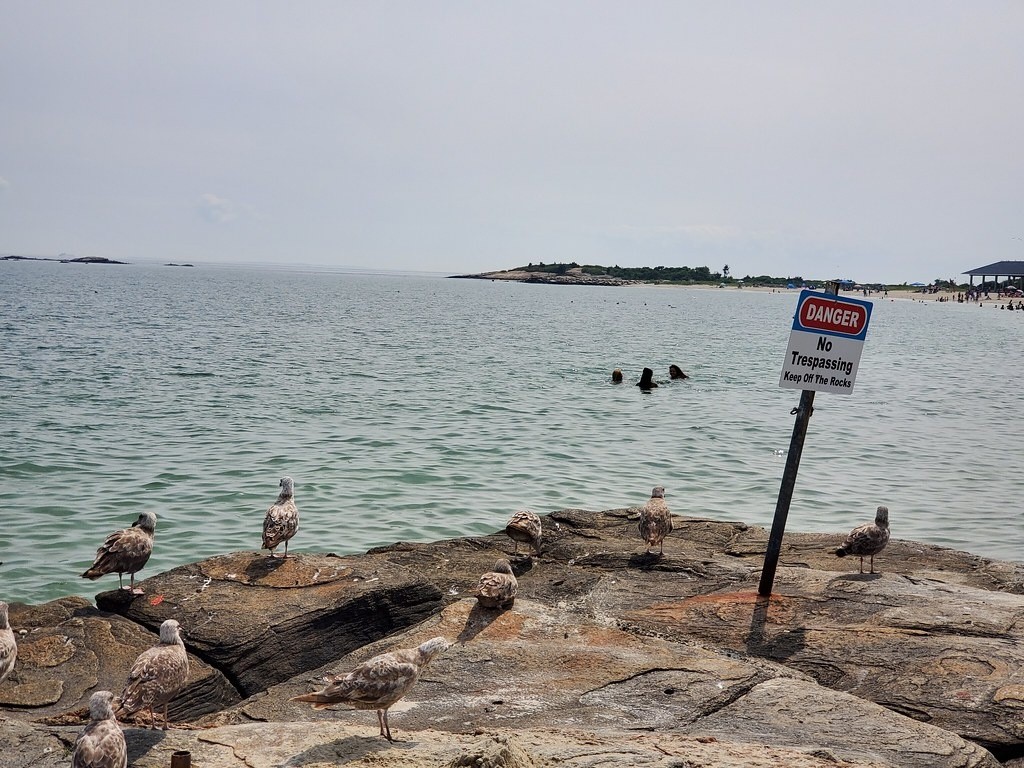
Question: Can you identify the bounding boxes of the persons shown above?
[669,365,689,380]
[636,368,658,388]
[612,369,623,382]
[487,270,1024,313]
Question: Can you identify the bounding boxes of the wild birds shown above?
[71,690,127,768]
[0,601,19,687]
[288,636,456,744]
[80,512,158,595]
[505,510,543,561]
[260,476,300,559]
[116,618,190,730]
[835,506,891,574]
[474,558,518,610]
[637,486,675,557]
